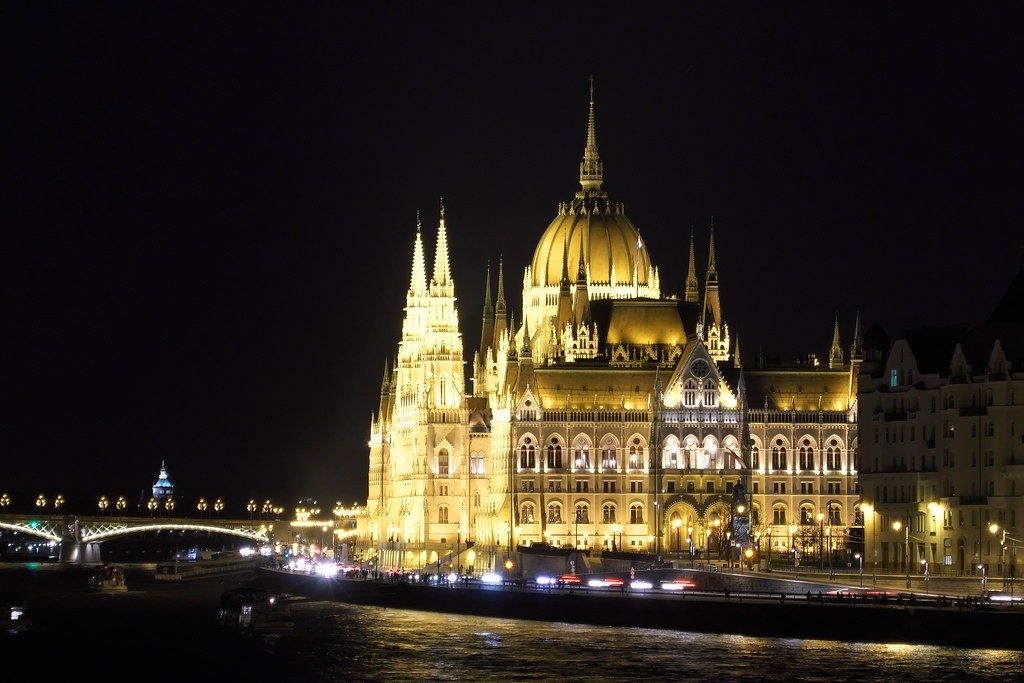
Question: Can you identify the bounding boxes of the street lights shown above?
[147,496,159,519]
[0,492,11,511]
[729,500,746,570]
[246,496,345,521]
[36,492,48,513]
[97,495,108,514]
[197,497,207,518]
[116,497,127,515]
[213,499,224,519]
[55,494,64,514]
[164,498,175,517]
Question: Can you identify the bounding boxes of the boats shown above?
[89,564,127,595]
[215,588,297,634]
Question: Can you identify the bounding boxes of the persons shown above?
[304,568,469,585]
[806,589,812,605]
[620,584,624,596]
[817,590,824,606]
[557,579,565,589]
[835,591,993,607]
